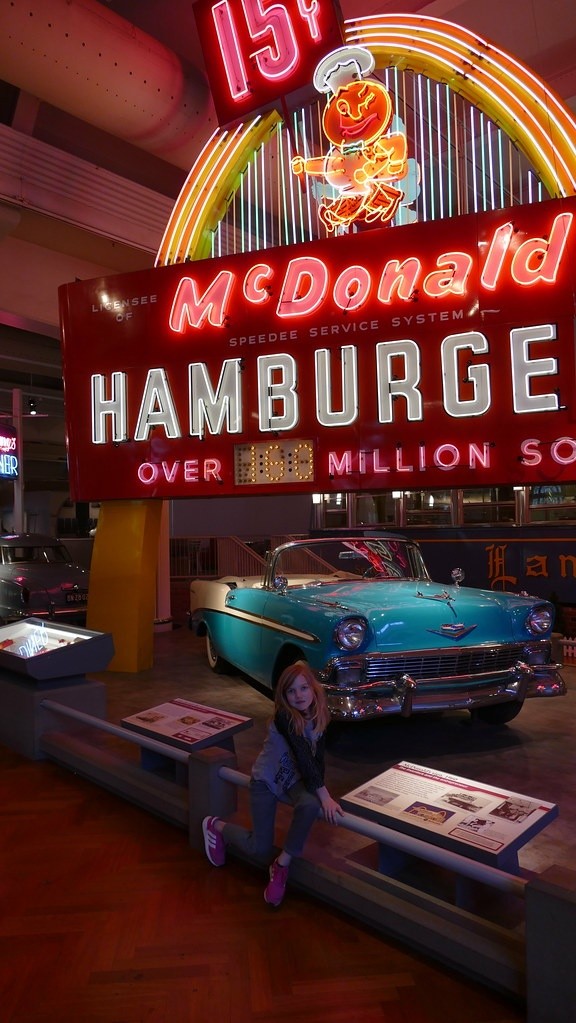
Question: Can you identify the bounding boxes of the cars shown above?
[1,532,90,628]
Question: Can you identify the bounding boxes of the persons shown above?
[202,666,345,908]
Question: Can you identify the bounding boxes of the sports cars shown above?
[191,525,568,754]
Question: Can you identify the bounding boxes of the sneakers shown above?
[203,815,226,866]
[265,857,290,907]
